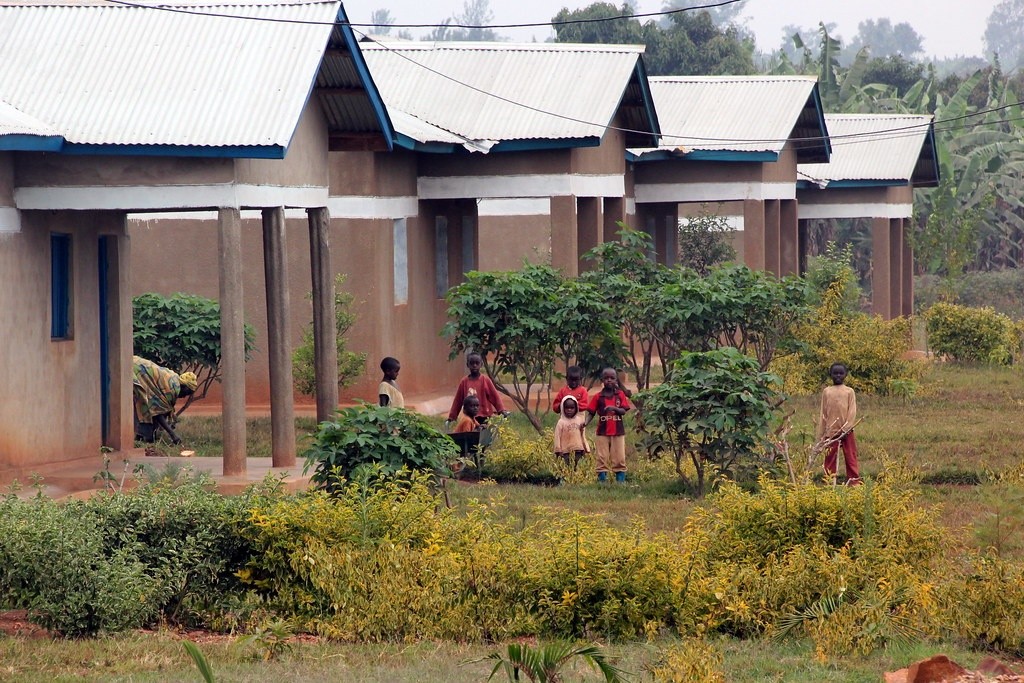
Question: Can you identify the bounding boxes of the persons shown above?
[378,356,405,438]
[448,352,508,467]
[452,394,488,435]
[816,363,863,485]
[554,395,590,473]
[552,365,591,423]
[579,366,631,483]
[132,355,197,446]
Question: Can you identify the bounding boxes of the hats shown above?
[179,372,198,391]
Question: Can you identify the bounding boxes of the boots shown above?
[617,472,624,481]
[598,472,606,480]
